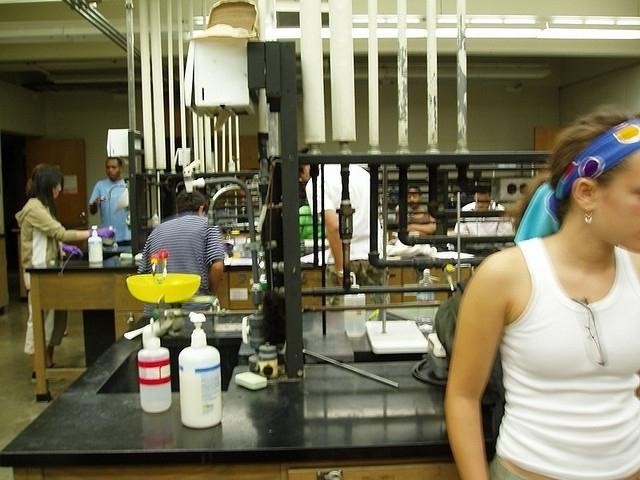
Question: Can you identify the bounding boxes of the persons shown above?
[451,181,516,237]
[89,155,133,249]
[442,106,639,480]
[295,149,388,310]
[394,184,439,244]
[15,161,115,381]
[132,189,228,315]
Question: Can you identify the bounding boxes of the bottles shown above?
[138,335,173,413]
[415,268,436,323]
[248,345,279,380]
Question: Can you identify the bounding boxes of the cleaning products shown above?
[88,224,104,264]
[177,311,224,429]
[416,268,438,301]
[345,271,366,338]
[137,318,175,414]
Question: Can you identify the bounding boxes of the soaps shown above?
[234,372,267,390]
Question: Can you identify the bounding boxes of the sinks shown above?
[97,336,242,391]
[58,251,123,261]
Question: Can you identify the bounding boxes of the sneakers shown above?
[32,364,65,383]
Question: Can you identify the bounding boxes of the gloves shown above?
[62,244,83,256]
[89,228,114,238]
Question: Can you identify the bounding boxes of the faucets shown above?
[105,184,128,200]
[183,160,265,352]
[208,184,261,226]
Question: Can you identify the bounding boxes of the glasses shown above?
[572,298,606,365]
